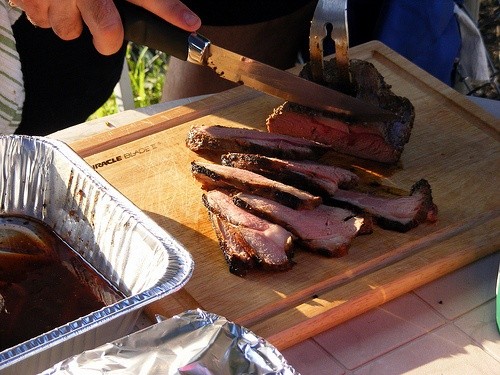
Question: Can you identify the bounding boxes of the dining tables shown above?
[42,94,500,375]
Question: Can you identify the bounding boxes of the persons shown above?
[1,0,304,138]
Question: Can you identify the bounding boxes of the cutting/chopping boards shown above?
[65,39,499,355]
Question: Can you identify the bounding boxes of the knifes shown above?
[83,0,405,122]
